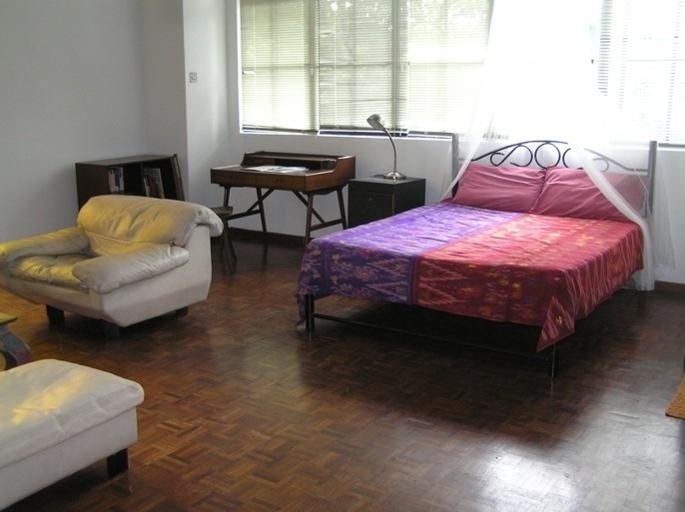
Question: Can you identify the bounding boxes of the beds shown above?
[296,141,656,389]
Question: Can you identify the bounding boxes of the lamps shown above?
[367,114,406,179]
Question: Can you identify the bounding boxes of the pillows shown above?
[445,161,545,214]
[530,167,650,218]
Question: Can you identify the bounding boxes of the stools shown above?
[210,206,236,276]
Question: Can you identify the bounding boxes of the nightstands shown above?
[349,175,426,227]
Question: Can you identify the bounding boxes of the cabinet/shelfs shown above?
[77,154,187,211]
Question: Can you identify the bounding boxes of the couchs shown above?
[0,359,143,509]
[0,195,223,337]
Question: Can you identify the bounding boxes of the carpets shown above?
[666,376,685,421]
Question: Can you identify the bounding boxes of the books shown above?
[139,164,167,198]
[241,165,309,174]
[108,165,124,193]
